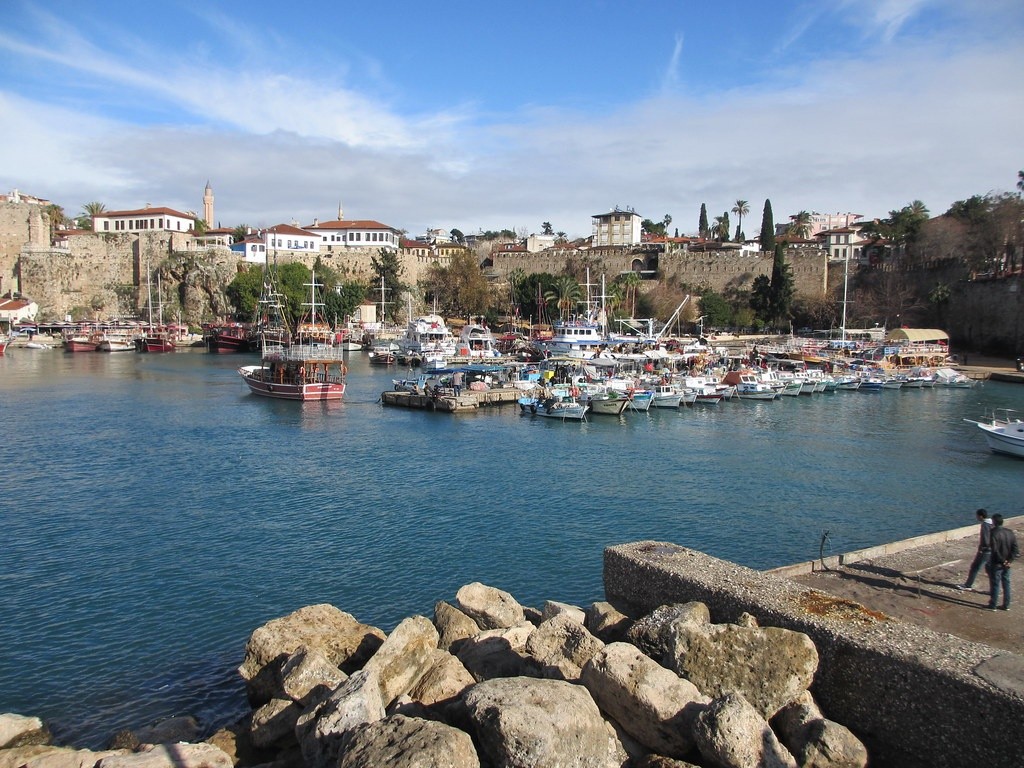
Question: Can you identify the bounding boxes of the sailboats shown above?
[363,276,399,347]
[295,270,333,348]
[237,346,347,402]
[247,226,295,352]
[133,261,176,353]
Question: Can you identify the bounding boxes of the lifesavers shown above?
[742,389,749,395]
[519,403,525,411]
[545,409,552,415]
[529,405,537,413]
[338,365,348,375]
[425,400,436,408]
[267,385,273,391]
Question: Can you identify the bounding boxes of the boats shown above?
[206,322,246,355]
[388,317,977,423]
[342,333,364,351]
[961,408,1024,459]
[99,333,135,352]
[368,347,395,364]
[0,341,12,353]
[61,330,99,352]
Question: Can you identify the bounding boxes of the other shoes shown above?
[1002,605,1011,610]
[957,584,972,591]
[982,606,997,612]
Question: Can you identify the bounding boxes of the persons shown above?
[956,509,994,591]
[983,514,1019,612]
[453,371,462,397]
[424,382,433,395]
[412,383,421,394]
